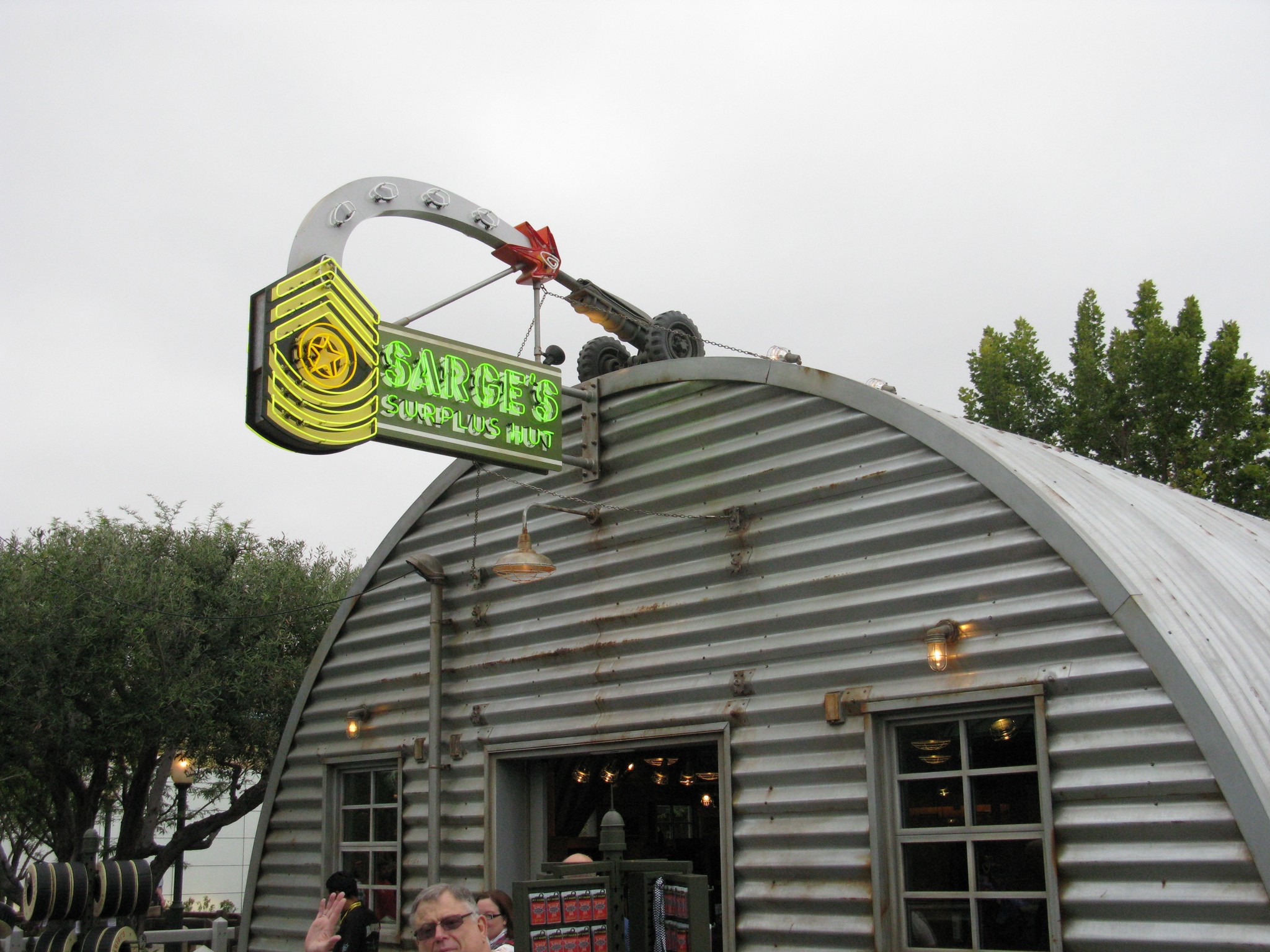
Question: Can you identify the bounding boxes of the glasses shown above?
[483,913,503,920]
[413,912,475,941]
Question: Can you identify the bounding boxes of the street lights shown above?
[162,748,196,952]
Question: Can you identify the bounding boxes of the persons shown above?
[324,870,382,952]
[475,889,517,952]
[302,883,491,952]
[151,876,166,910]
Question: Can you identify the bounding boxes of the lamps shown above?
[925,617,961,672]
[346,706,371,740]
[569,751,720,808]
[911,715,1024,799]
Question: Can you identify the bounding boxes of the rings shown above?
[322,914,326,918]
